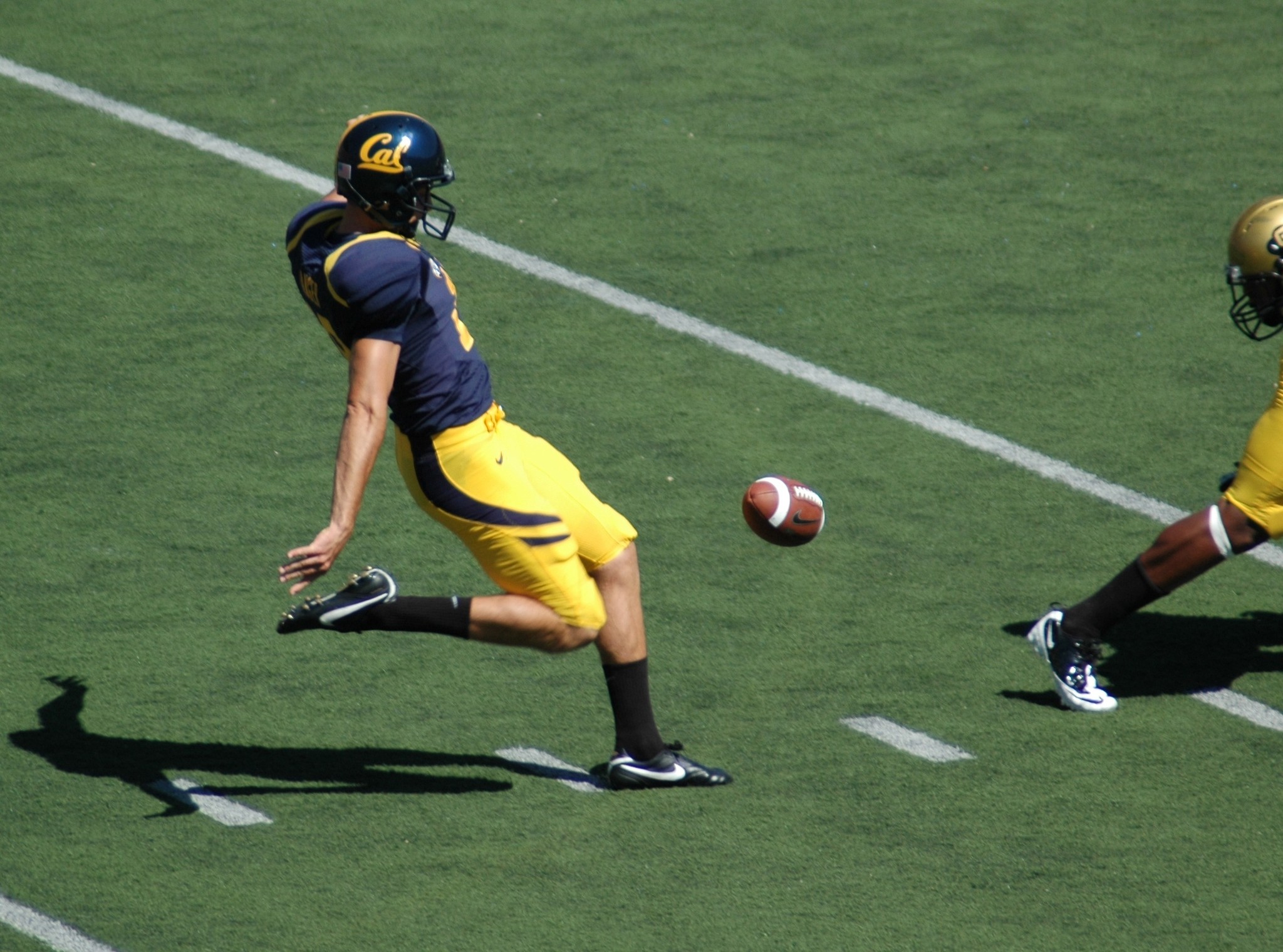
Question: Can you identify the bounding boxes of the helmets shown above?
[1224,193,1283,345]
[333,108,457,240]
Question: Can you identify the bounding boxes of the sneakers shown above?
[275,565,399,635]
[1026,600,1118,714]
[606,744,733,790]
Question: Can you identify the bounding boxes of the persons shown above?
[1027,194,1283,710]
[276,110,733,790]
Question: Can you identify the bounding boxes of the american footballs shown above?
[742,476,825,547]
